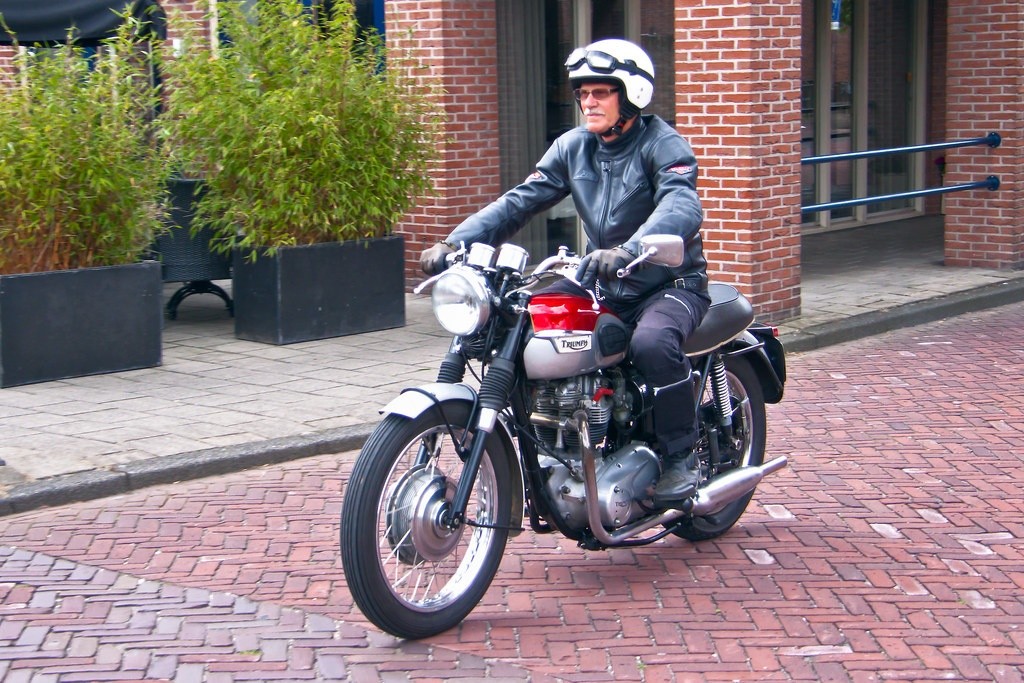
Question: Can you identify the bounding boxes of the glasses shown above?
[563,47,654,86]
[573,87,620,100]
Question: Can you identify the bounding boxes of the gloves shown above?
[420,240,457,276]
[575,245,639,288]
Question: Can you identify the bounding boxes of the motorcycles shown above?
[340,232,789,640]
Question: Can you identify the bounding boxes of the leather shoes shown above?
[656,452,701,500]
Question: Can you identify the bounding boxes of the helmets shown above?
[568,39,655,109]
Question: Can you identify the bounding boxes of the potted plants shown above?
[154,1,451,349]
[146,15,234,319]
[0,0,166,389]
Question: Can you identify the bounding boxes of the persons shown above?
[418,39,714,502]
